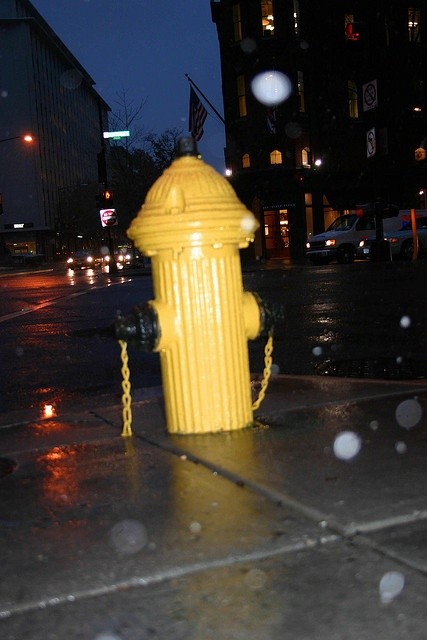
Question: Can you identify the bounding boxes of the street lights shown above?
[103,189,113,202]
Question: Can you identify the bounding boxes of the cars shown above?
[65,249,94,270]
[93,251,113,268]
[110,246,142,268]
[382,218,427,267]
[305,214,378,265]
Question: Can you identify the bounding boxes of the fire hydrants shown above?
[109,136,285,437]
